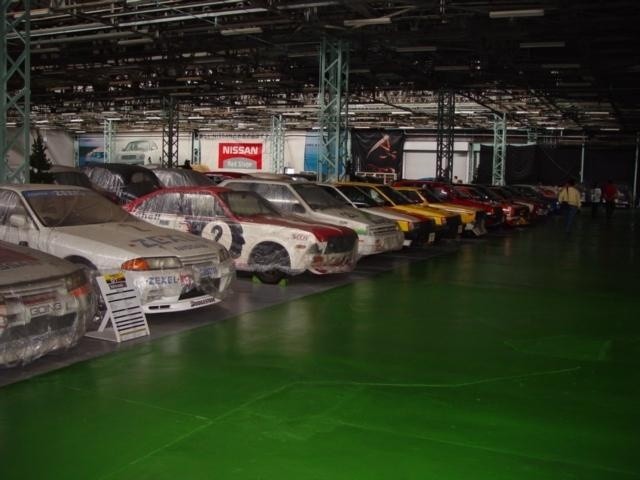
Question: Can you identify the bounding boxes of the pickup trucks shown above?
[85,139,165,166]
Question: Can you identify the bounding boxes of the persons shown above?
[451,176,460,184]
[603,178,619,224]
[558,177,581,241]
[621,184,633,210]
[176,159,193,170]
[589,180,603,222]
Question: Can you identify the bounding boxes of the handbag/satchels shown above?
[558,186,570,212]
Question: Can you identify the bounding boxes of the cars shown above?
[217,178,405,262]
[600,186,630,209]
[0,239,100,371]
[0,182,237,318]
[121,186,359,285]
[78,164,562,253]
